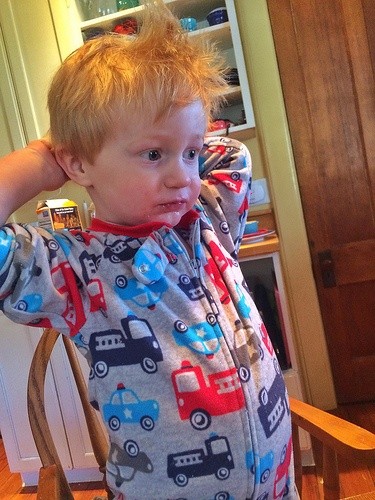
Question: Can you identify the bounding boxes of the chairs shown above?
[27,326,375,500]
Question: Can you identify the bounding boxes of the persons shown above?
[0,0,299,500]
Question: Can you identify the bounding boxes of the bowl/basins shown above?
[180,18,197,31]
[245,222,259,233]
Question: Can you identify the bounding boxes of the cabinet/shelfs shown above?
[69,0,257,137]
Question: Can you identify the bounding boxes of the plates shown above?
[240,230,275,244]
